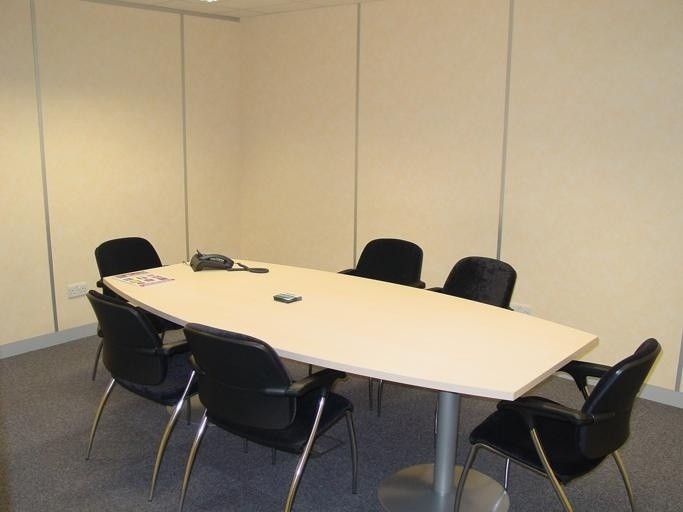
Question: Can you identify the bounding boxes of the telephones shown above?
[190,249,234,272]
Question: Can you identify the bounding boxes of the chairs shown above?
[91,235,184,381]
[378,255,518,435]
[307,238,426,411]
[454,337,661,511]
[85,290,202,501]
[178,321,358,512]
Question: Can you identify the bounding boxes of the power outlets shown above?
[67,281,88,299]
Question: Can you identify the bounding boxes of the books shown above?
[112,270,175,288]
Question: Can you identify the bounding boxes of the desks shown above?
[102,258,600,511]
